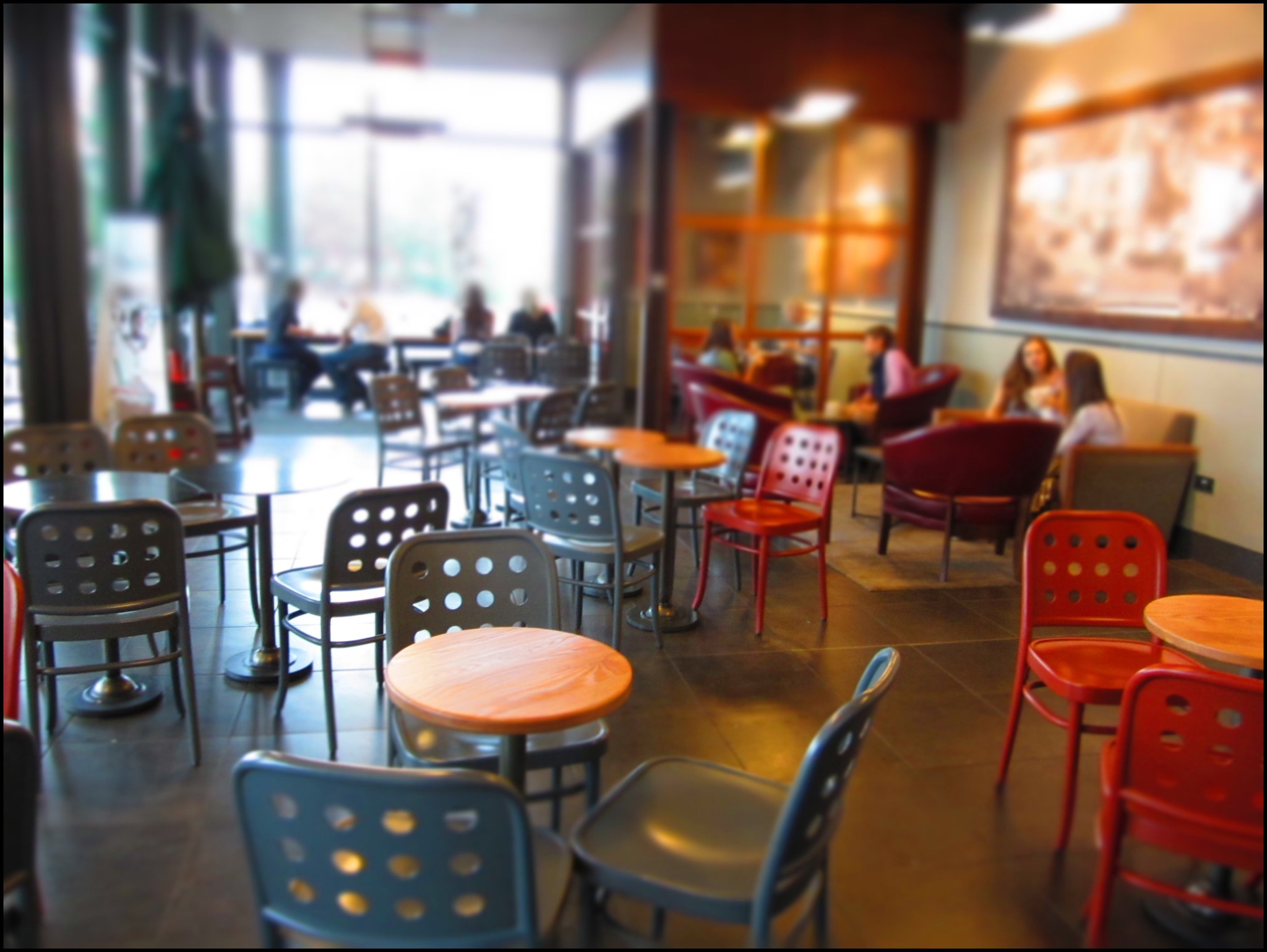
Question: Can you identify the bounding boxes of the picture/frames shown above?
[987,54,1266,342]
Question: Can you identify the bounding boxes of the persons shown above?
[697,315,742,377]
[122,303,146,342]
[324,299,392,411]
[451,283,496,379]
[1050,348,1131,459]
[762,297,822,391]
[263,276,325,412]
[864,324,923,403]
[986,334,1070,430]
[507,287,556,432]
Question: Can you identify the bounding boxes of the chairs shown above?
[0,317,1266,952]
